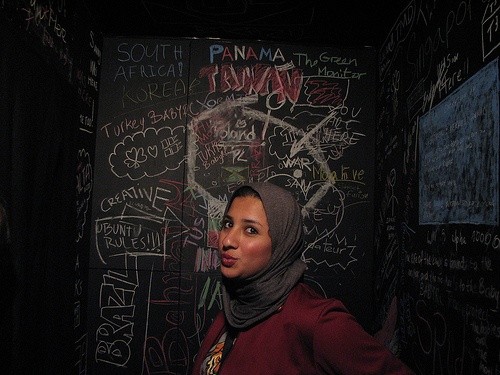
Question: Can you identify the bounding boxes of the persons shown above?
[194,182,417,375]
